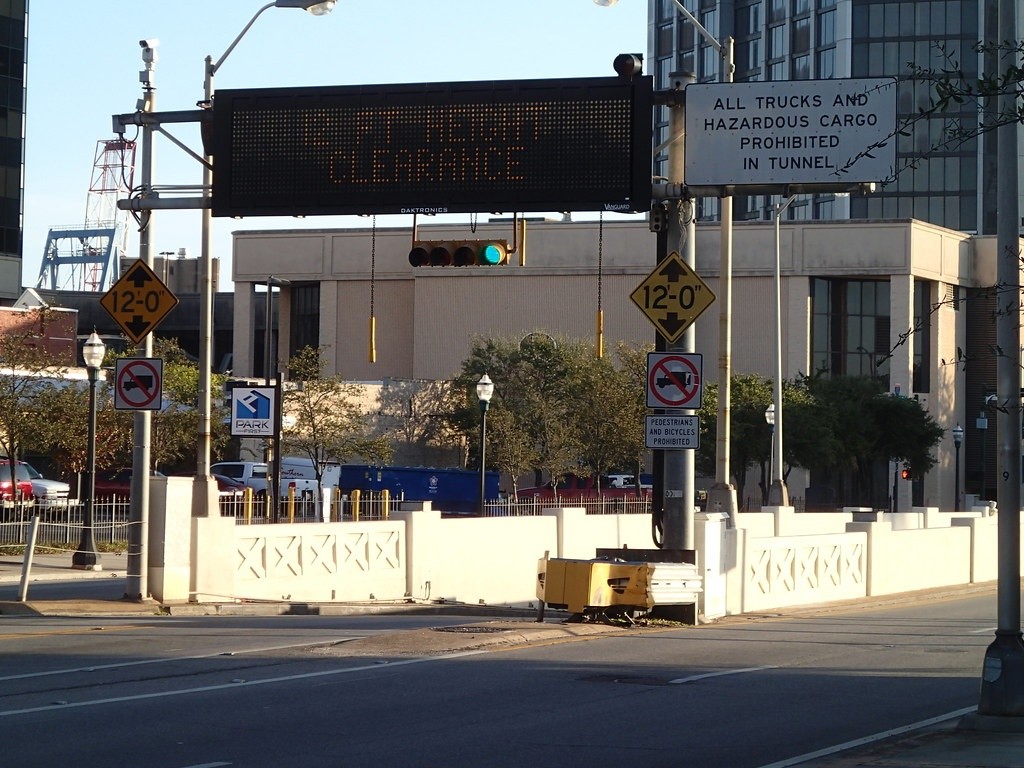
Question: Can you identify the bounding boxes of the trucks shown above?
[208,462,320,515]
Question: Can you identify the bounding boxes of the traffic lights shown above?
[901,470,911,479]
[408,238,510,268]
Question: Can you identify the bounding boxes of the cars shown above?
[170,470,248,512]
[22,462,79,518]
[92,467,166,511]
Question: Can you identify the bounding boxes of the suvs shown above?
[0,457,36,522]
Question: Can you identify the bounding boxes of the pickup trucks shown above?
[507,472,654,519]
[606,475,652,489]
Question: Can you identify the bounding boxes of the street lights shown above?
[71,323,103,570]
[951,421,965,512]
[196,0,331,519]
[475,372,495,518]
[765,403,775,505]
[976,409,988,501]
